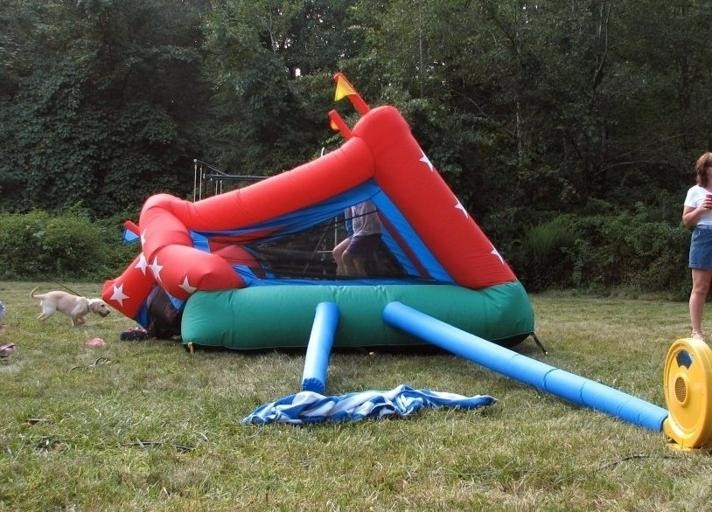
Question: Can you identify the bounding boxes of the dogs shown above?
[30,286,111,328]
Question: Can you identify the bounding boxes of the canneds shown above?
[706,195,712,209]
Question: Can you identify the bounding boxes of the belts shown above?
[695,225,712,230]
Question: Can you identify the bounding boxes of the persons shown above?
[332,198,383,279]
[683,152,712,342]
